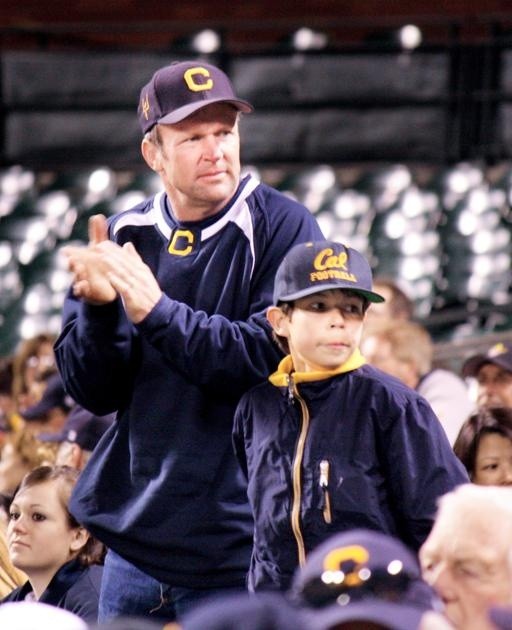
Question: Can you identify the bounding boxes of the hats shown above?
[37,404,117,448]
[182,592,298,627]
[272,240,385,307]
[287,530,431,628]
[18,378,76,417]
[461,342,510,381]
[136,61,253,135]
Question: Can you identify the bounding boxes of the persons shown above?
[0,332,108,630]
[52,60,326,630]
[173,484,512,630]
[232,241,471,598]
[366,279,512,488]
